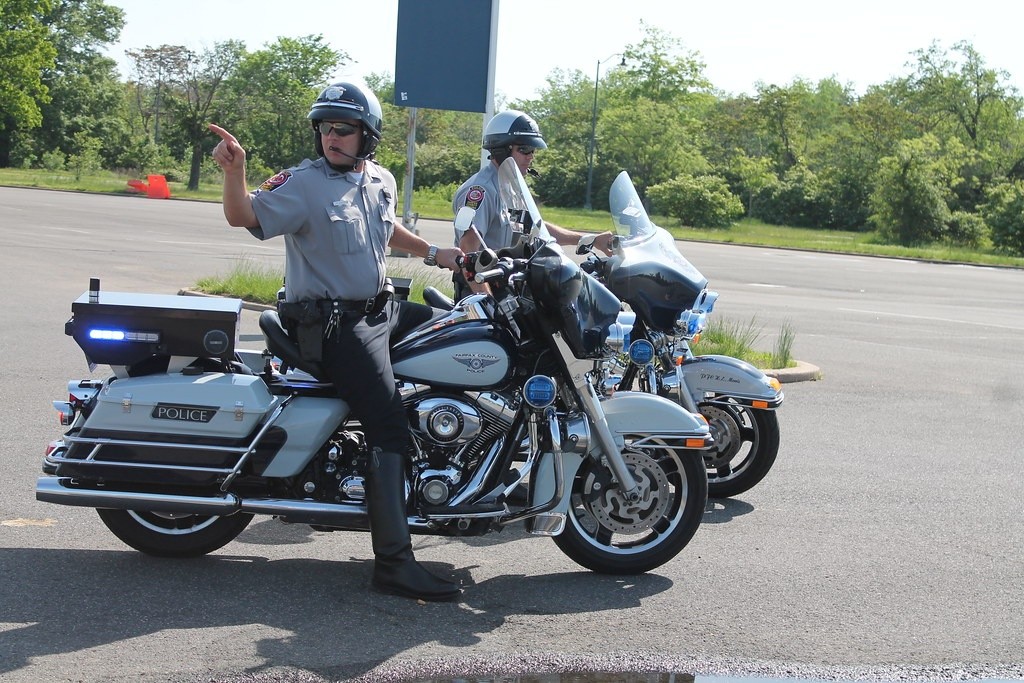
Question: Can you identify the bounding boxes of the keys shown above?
[325,314,340,343]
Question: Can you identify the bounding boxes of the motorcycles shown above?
[34,154,785,574]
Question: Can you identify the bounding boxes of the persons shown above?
[209,79,462,601]
[452,110,614,308]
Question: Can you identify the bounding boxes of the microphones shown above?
[526,167,539,178]
[328,132,380,161]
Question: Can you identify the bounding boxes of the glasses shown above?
[316,121,360,137]
[511,146,536,155]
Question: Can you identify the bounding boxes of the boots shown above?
[365,451,463,601]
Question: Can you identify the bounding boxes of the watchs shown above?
[423,245,439,266]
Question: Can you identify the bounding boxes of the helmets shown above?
[482,110,549,150]
[307,81,384,159]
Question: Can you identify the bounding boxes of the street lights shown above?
[582,52,628,209]
